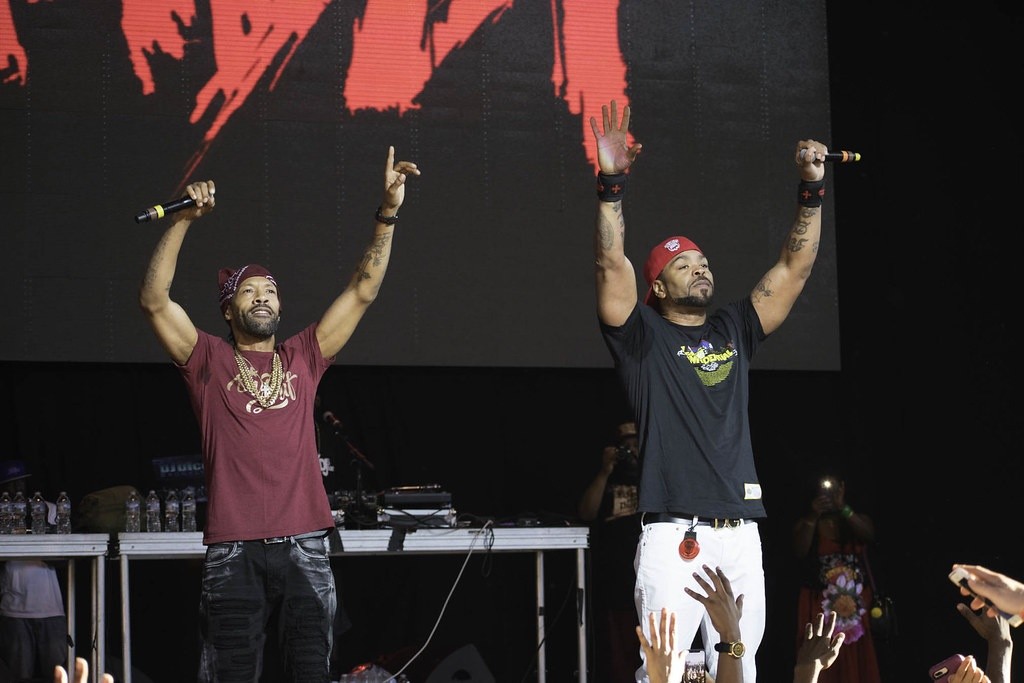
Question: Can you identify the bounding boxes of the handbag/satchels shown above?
[870,584,899,647]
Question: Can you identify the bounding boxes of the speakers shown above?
[383,642,496,683]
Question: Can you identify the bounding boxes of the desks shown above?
[0,533,111,683]
[93,527,591,683]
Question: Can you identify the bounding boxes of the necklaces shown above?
[233,349,283,407]
[315,419,321,454]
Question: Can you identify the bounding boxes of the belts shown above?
[261,528,328,544]
[643,511,755,530]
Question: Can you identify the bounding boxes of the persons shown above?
[590,101,828,682]
[0,459,68,683]
[636,565,845,682]
[946,564,1024,683]
[791,474,880,683]
[314,388,349,511]
[572,422,642,683]
[686,666,703,683]
[137,146,421,683]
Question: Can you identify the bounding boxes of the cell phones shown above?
[929,653,965,683]
[948,567,1023,627]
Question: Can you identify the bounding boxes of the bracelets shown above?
[376,206,399,224]
[597,171,625,202]
[798,179,824,207]
[840,504,854,519]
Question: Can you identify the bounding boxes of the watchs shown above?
[715,639,745,658]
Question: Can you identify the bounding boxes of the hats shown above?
[643,236,704,305]
[216,264,279,325]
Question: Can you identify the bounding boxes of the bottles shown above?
[361,675,370,683]
[31,493,46,534]
[195,487,207,532]
[56,492,71,534]
[179,487,196,532]
[0,492,13,534]
[350,674,361,683]
[399,675,410,683]
[340,674,349,683]
[165,491,179,532]
[12,492,27,534]
[125,492,140,532]
[146,491,160,532]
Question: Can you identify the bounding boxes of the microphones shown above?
[799,148,862,163]
[136,192,213,223]
[323,411,341,427]
